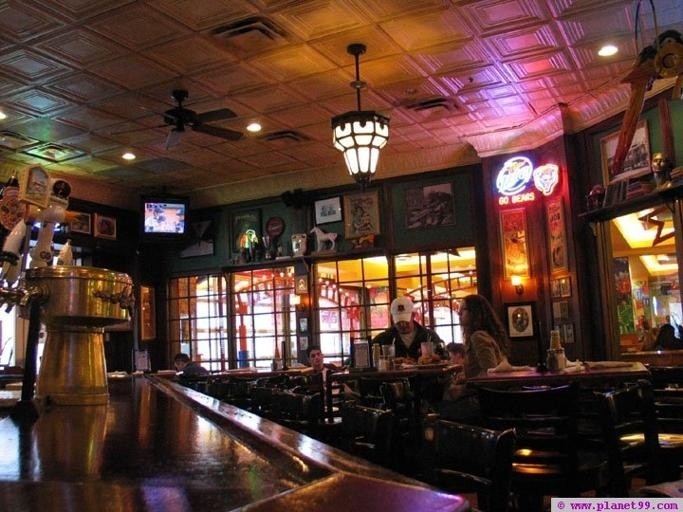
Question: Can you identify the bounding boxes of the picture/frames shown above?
[340,186,384,241]
[496,207,531,282]
[66,210,91,234]
[543,196,569,275]
[503,301,539,341]
[549,276,574,345]
[92,211,117,239]
[175,218,218,261]
[299,316,306,333]
[311,193,343,226]
[597,119,653,187]
[402,178,457,231]
[293,273,308,296]
[227,206,266,253]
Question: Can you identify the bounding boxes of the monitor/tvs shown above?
[141,200,190,238]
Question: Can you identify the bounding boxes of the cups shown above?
[421,342,432,364]
[546,349,566,370]
[382,345,396,368]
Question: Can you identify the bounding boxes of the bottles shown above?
[549,330,560,349]
[372,343,381,367]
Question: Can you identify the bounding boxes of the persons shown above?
[173,353,209,390]
[440,296,510,422]
[637,314,683,351]
[446,342,467,385]
[146,208,184,234]
[302,347,349,396]
[370,298,444,358]
[650,152,674,188]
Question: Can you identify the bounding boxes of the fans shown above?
[108,86,245,153]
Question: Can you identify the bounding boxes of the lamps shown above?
[508,276,524,294]
[328,44,391,186]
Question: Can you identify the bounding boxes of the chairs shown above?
[150,347,683,512]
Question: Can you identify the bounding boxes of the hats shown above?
[389,296,416,324]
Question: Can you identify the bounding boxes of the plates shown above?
[417,363,448,369]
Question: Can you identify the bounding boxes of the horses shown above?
[310,225,341,252]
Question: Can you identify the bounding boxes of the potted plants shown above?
[618,303,636,351]
